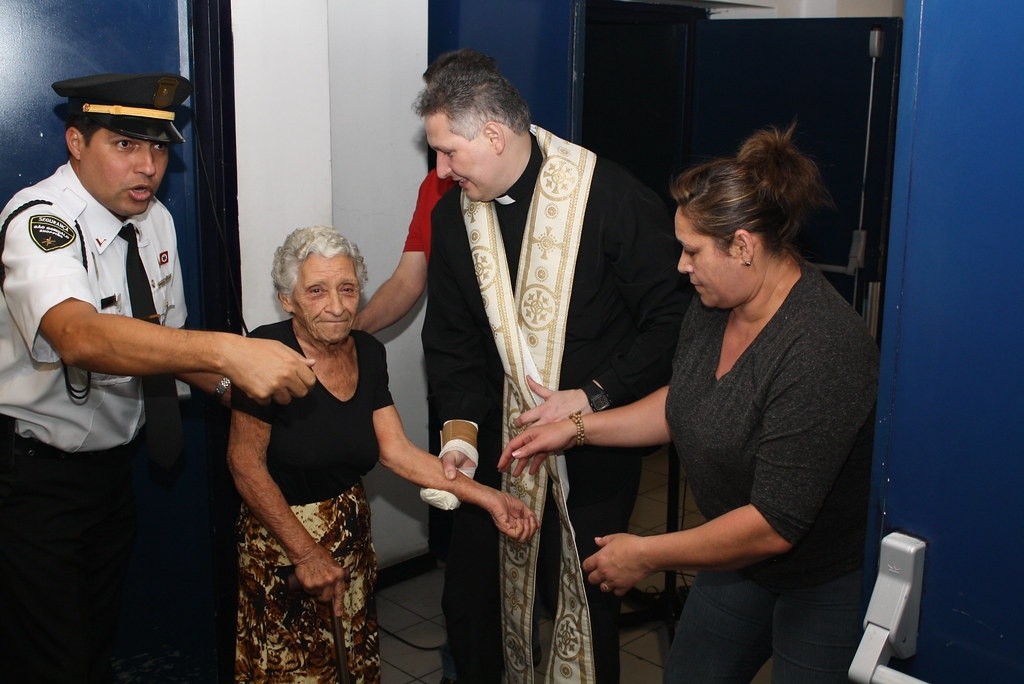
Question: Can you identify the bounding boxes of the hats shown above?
[51,72,193,143]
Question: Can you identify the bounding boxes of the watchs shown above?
[580,380,612,413]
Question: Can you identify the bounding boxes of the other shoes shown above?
[532,607,542,668]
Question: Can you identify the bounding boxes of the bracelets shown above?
[570,413,585,445]
[213,376,231,398]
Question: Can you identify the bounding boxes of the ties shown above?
[119,222,184,470]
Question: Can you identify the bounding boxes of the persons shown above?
[227,224,540,684]
[495,127,877,684]
[0,73,316,684]
[352,48,541,684]
[412,61,690,684]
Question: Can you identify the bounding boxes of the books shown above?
[860,280,881,342]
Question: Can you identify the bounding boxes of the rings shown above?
[602,584,611,592]
